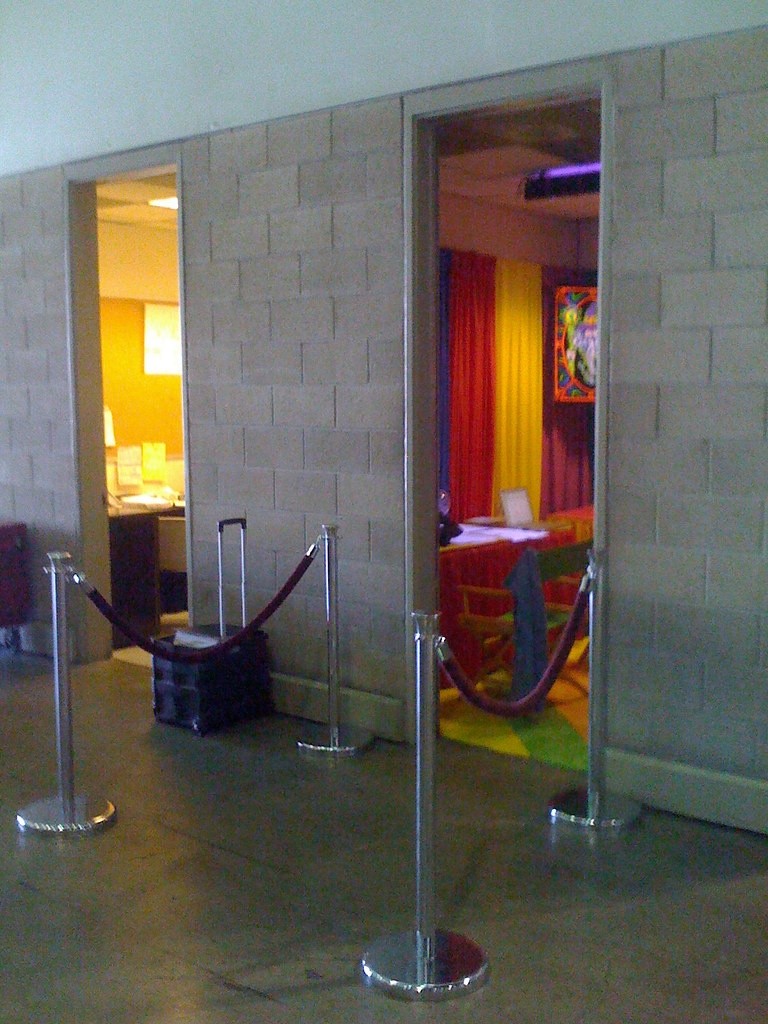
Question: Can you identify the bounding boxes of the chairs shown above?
[457,576,586,696]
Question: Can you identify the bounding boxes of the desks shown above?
[440,503,593,703]
[109,493,189,650]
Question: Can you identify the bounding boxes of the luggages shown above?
[154,518,276,734]
